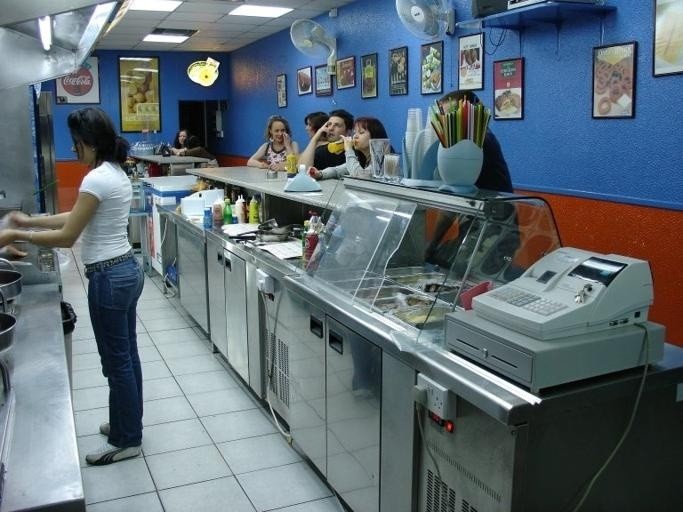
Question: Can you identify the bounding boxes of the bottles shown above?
[301,210,319,272]
[286,151,297,178]
[204,194,258,230]
[328,137,356,154]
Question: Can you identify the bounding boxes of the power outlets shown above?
[255,268,274,295]
[417,373,457,422]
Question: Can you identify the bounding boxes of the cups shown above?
[384,153,400,180]
[405,107,440,180]
[369,138,391,179]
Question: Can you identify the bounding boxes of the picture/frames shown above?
[276,74,288,108]
[335,56,356,90]
[388,46,408,96]
[297,65,313,96]
[315,64,333,97]
[420,40,444,95]
[360,52,377,99]
[117,55,161,132]
[458,32,485,91]
[492,56,524,120]
[54,55,101,105]
[592,40,637,119]
[653,0,683,77]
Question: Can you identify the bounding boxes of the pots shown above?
[229,218,292,242]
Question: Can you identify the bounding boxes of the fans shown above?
[186,57,221,87]
[395,0,454,40]
[290,18,337,76]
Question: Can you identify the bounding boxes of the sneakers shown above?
[99,421,111,436]
[85,443,142,466]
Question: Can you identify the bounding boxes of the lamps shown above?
[37,15,52,53]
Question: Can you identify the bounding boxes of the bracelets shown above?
[29,230,33,243]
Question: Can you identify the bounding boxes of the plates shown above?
[220,223,261,237]
[495,88,521,118]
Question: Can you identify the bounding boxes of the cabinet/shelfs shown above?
[287,285,382,512]
[206,236,250,388]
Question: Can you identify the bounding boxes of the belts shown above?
[86,249,133,273]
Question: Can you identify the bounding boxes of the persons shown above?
[0,211,28,260]
[170,128,190,155]
[247,115,299,171]
[0,106,144,466]
[172,136,220,168]
[431,89,521,275]
[304,112,329,138]
[307,117,396,179]
[297,109,368,173]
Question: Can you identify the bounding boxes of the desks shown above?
[128,154,212,176]
